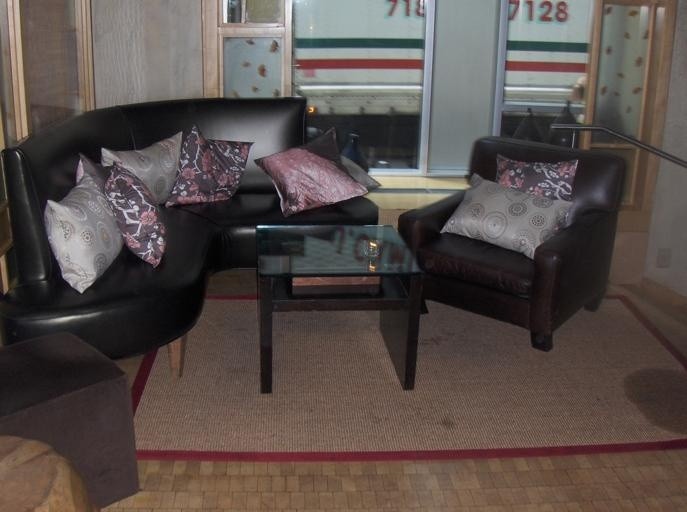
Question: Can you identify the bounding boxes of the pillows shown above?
[100,131,183,206]
[340,155,382,192]
[75,153,100,184]
[254,125,369,219]
[495,153,580,201]
[164,122,256,208]
[439,173,574,261]
[104,161,168,269]
[43,171,125,295]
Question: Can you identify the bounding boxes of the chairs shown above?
[398,136,626,351]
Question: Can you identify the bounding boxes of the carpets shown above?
[131,294,687,463]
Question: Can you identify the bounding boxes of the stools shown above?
[0,332,140,512]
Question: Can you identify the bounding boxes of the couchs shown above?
[0,96,378,361]
